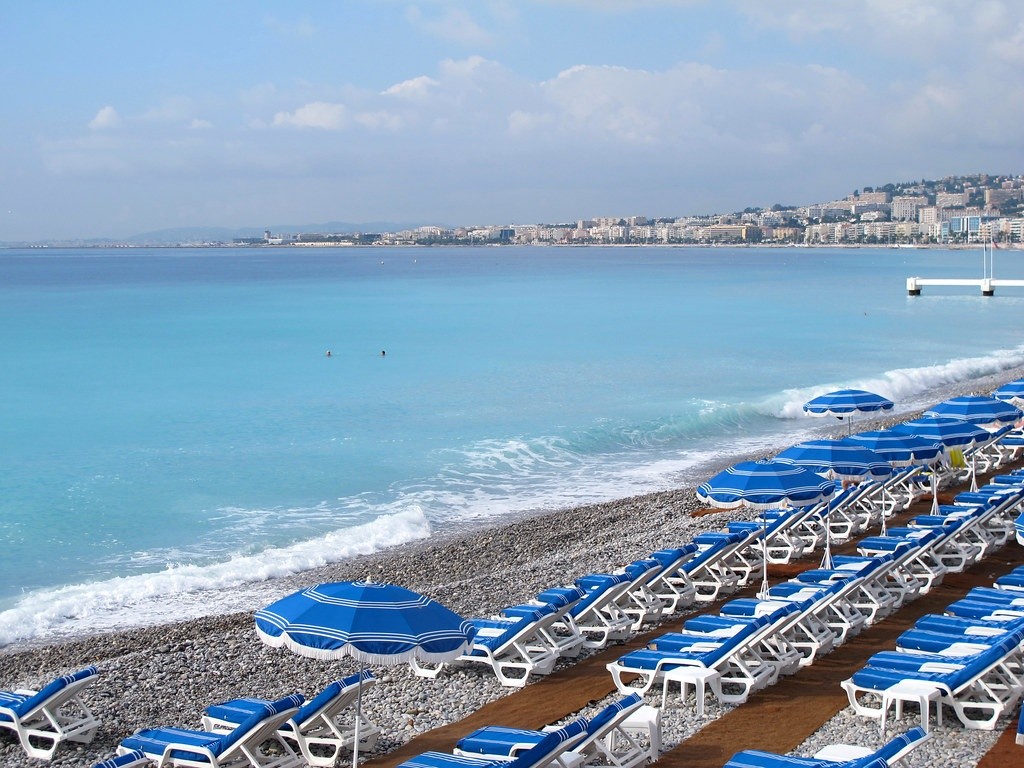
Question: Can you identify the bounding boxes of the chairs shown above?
[0,665,103,760]
[90,424,1024,768]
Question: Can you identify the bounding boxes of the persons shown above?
[834,480,849,497]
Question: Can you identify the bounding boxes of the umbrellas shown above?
[252,576,480,768]
[695,380,1024,601]
